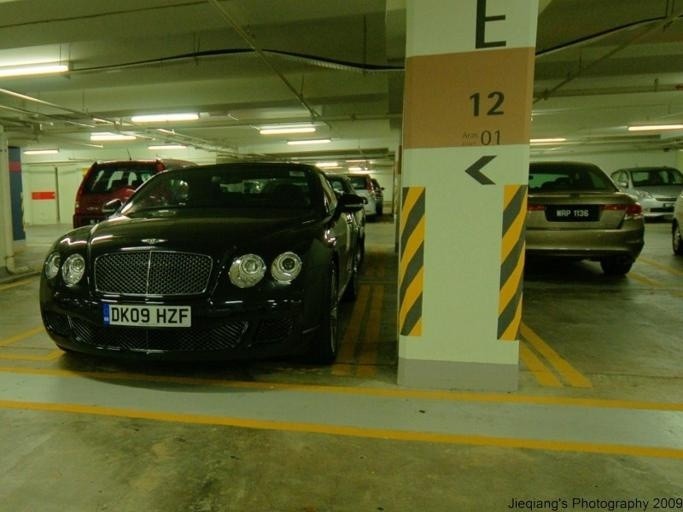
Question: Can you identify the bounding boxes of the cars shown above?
[38,161,361,363]
[526,161,645,274]
[72,159,199,228]
[344,173,378,222]
[669,191,683,255]
[609,166,683,221]
[372,179,385,215]
[326,174,368,267]
